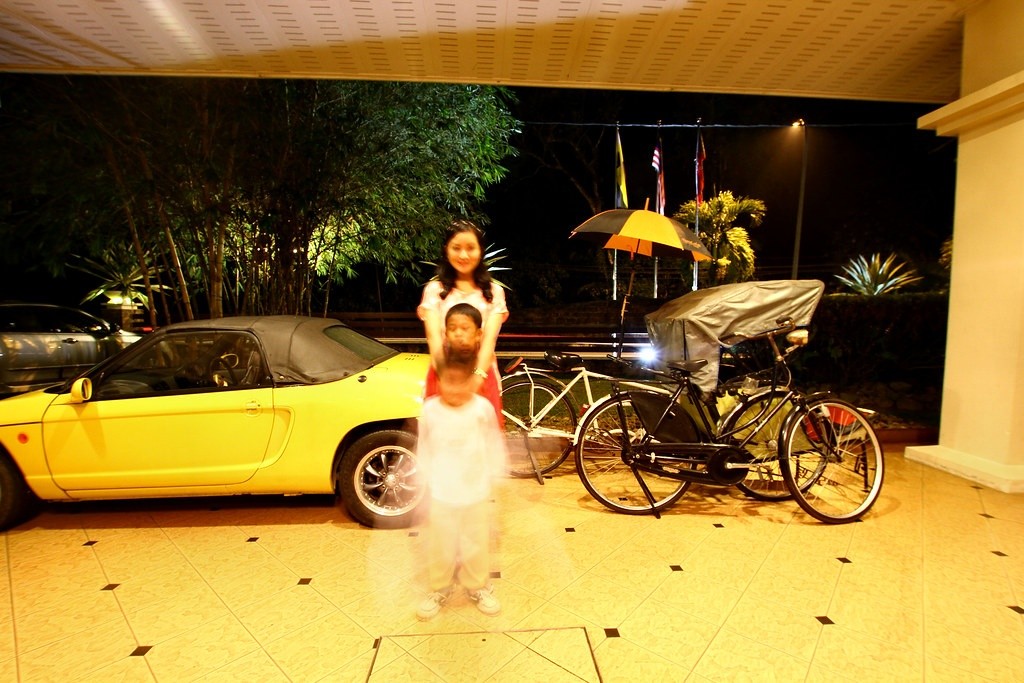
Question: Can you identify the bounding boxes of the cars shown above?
[0,303,176,402]
[0,315,431,533]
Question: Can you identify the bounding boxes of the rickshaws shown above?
[644,279,878,503]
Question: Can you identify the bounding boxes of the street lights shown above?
[792,118,806,280]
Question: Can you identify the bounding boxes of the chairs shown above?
[238,343,262,385]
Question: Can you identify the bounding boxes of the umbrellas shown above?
[567,198,716,357]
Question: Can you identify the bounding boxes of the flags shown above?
[615,129,628,208]
[651,127,665,217]
[695,130,706,205]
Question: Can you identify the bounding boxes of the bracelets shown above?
[473,367,488,380]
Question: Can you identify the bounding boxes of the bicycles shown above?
[572,316,886,525]
[497,352,680,485]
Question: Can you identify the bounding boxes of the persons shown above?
[412,355,508,620]
[416,221,508,399]
[424,304,505,592]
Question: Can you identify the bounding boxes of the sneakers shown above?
[414,583,457,619]
[468,584,501,616]
